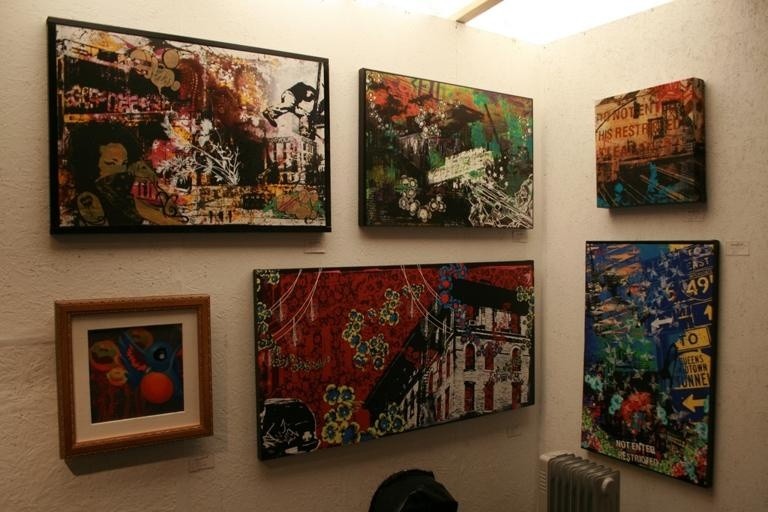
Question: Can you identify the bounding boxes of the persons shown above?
[65,118,188,226]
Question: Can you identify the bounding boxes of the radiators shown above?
[534,453,621,512]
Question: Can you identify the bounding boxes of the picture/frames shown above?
[251,258,539,462]
[359,67,534,230]
[45,11,336,233]
[53,291,215,464]
[578,235,720,492]
